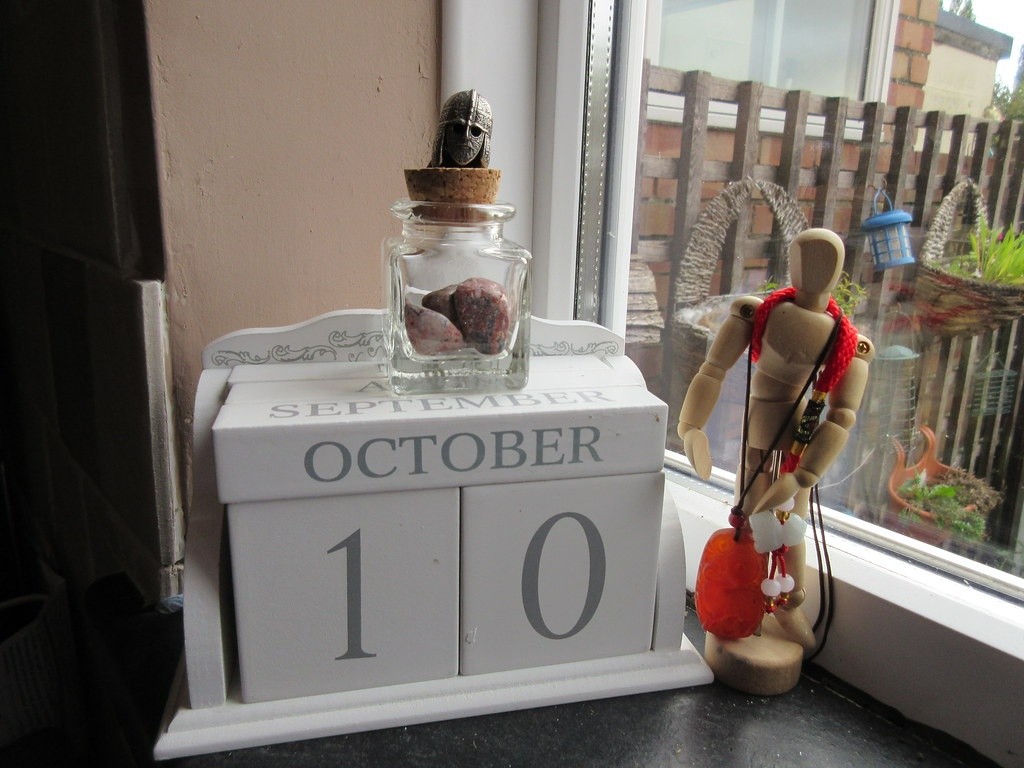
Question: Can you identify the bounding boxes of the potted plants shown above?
[888,424,1005,548]
[669,272,867,403]
[913,219,1023,338]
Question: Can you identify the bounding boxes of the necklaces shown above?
[693,285,858,640]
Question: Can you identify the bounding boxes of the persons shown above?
[677,226,876,649]
[429,89,492,167]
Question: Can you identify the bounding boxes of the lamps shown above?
[860,190,914,275]
[873,310,918,455]
